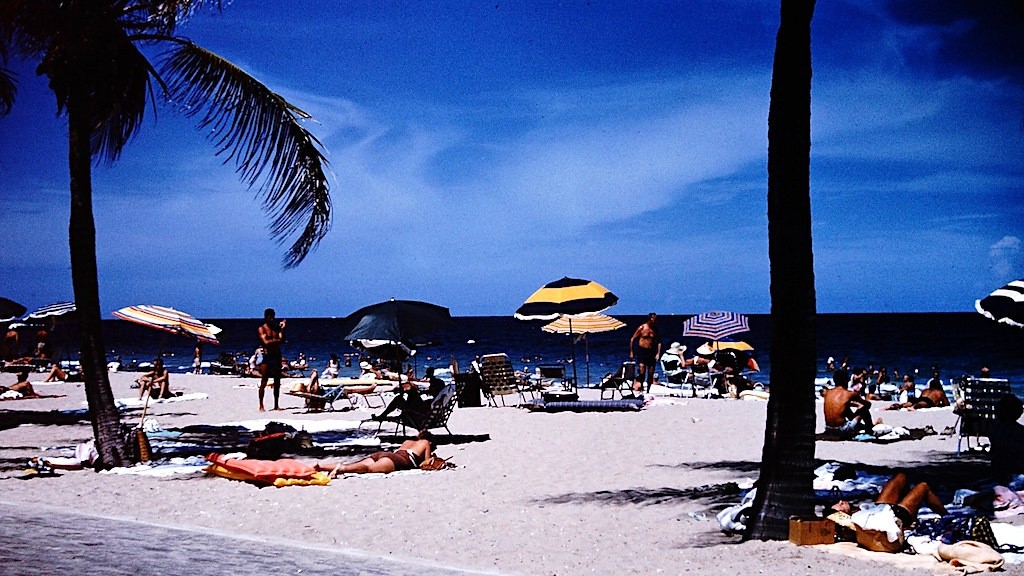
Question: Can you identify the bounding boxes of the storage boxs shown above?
[788,516,836,547]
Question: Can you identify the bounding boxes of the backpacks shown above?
[968,517,1001,554]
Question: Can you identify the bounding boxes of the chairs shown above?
[283,352,738,443]
[954,378,1012,455]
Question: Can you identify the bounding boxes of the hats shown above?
[696,341,716,355]
[360,361,372,369]
[666,342,687,355]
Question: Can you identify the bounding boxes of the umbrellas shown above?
[682,310,750,350]
[110,304,220,428]
[975,279,1024,327]
[342,296,453,397]
[349,337,415,363]
[513,276,626,397]
[9,301,77,330]
[696,336,753,354]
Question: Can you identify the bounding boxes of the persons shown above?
[245,345,554,426]
[316,431,436,473]
[193,339,202,374]
[257,308,286,412]
[0,327,85,398]
[138,357,171,400]
[629,313,662,392]
[651,342,755,393]
[823,471,950,553]
[744,352,760,372]
[824,356,989,437]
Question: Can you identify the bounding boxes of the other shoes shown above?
[940,427,956,435]
[923,426,937,435]
